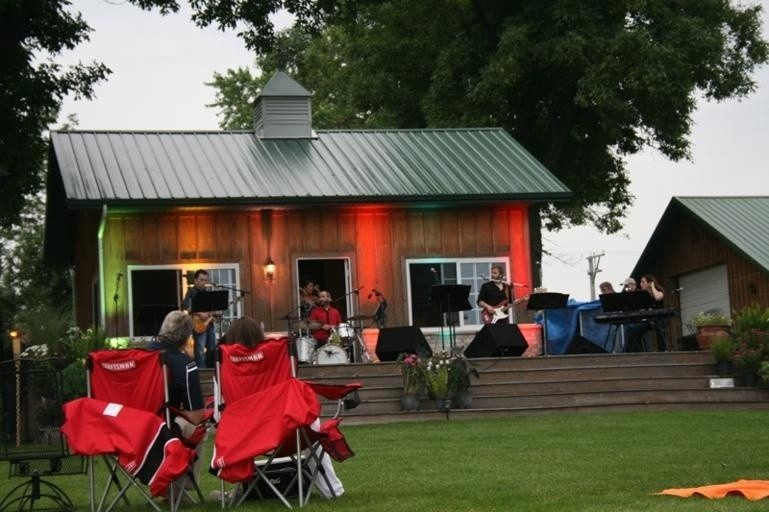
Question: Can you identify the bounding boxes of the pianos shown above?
[592,308,675,324]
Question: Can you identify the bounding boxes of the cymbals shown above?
[294,320,322,329]
[345,315,375,319]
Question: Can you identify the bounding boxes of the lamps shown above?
[264,257,277,282]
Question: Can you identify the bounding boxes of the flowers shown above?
[396,345,478,398]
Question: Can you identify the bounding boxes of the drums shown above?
[296,334,317,363]
[338,323,355,340]
[327,327,348,347]
[312,344,348,365]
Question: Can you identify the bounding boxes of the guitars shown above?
[192,292,249,333]
[482,288,546,324]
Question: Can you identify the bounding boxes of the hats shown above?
[619,278,636,285]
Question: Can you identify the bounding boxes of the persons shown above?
[599,283,617,294]
[301,279,322,319]
[631,273,670,352]
[310,290,342,350]
[225,316,346,501]
[182,268,219,370]
[476,266,522,325]
[145,310,210,507]
[618,277,654,352]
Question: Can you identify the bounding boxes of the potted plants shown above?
[684,302,769,387]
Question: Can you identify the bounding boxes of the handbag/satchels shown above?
[241,454,312,500]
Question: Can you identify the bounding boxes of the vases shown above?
[402,390,473,413]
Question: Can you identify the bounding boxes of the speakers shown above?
[463,323,530,358]
[375,326,433,361]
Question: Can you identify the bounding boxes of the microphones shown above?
[481,273,489,280]
[430,267,436,273]
[672,286,684,294]
